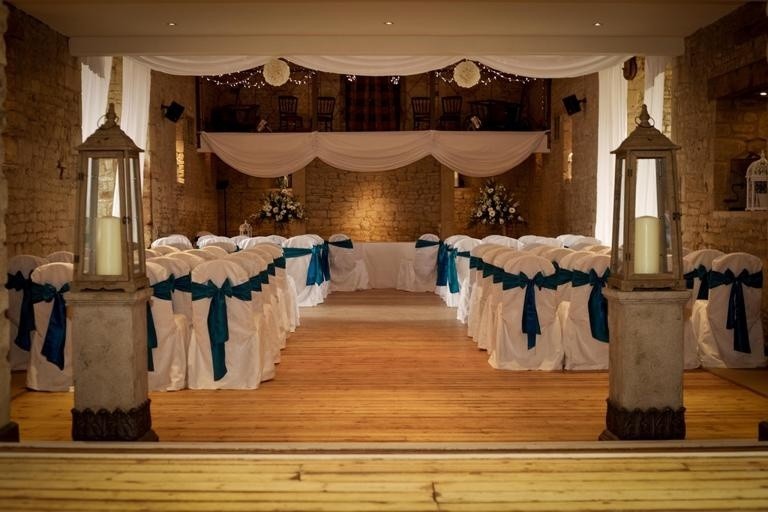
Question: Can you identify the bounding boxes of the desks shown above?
[214,104,259,132]
[467,99,523,130]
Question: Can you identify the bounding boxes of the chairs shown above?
[558,252,594,304]
[133,241,227,268]
[522,243,612,259]
[316,96,334,130]
[434,235,481,307]
[439,96,462,131]
[482,233,585,248]
[222,256,280,364]
[488,255,561,371]
[151,234,287,252]
[24,262,74,393]
[686,250,727,300]
[135,260,190,389]
[602,248,624,275]
[668,246,695,273]
[148,257,192,324]
[230,251,286,349]
[328,234,372,292]
[467,248,516,337]
[4,255,49,371]
[694,251,764,369]
[278,95,304,131]
[411,97,432,129]
[254,245,300,333]
[242,248,290,339]
[282,234,330,307]
[396,234,441,293]
[557,255,609,370]
[188,259,275,390]
[456,244,505,324]
[48,251,74,264]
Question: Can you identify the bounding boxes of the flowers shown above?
[250,183,308,225]
[463,176,528,230]
[264,60,290,86]
[453,60,480,88]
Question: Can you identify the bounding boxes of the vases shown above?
[483,222,507,236]
[274,221,289,237]
